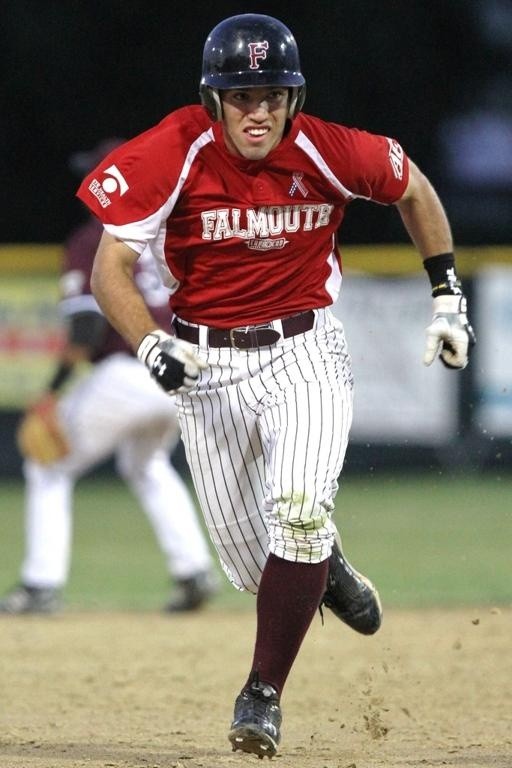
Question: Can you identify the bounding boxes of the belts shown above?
[173,309,315,349]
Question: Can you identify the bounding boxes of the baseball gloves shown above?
[16,394,69,467]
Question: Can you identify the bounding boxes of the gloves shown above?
[135,328,209,397]
[422,293,478,371]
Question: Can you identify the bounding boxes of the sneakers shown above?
[316,522,384,638]
[227,680,285,760]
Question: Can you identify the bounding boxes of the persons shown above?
[1,136,224,616]
[75,12,474,760]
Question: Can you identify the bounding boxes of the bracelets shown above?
[422,253,465,297]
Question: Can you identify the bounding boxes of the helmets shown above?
[199,13,306,90]
